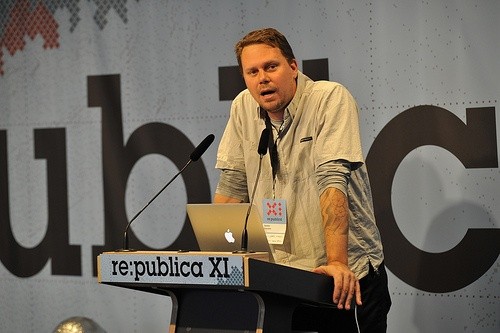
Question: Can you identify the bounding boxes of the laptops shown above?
[185,202,270,253]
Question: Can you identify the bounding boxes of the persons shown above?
[269,119,284,136]
[211,27,391,333]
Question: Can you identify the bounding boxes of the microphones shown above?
[115,134,216,250]
[242,128,271,252]
